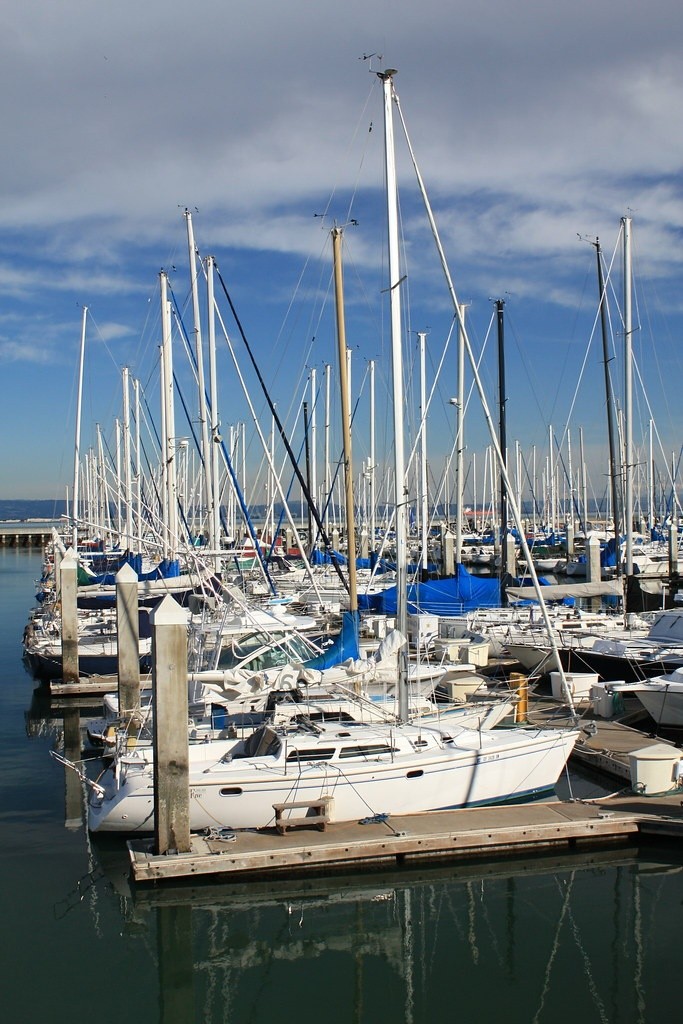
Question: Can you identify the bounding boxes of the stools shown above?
[273,801,328,835]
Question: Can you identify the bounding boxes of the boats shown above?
[20,53,683,835]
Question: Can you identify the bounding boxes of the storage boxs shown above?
[628,743,682,795]
[305,600,491,703]
[549,672,624,718]
